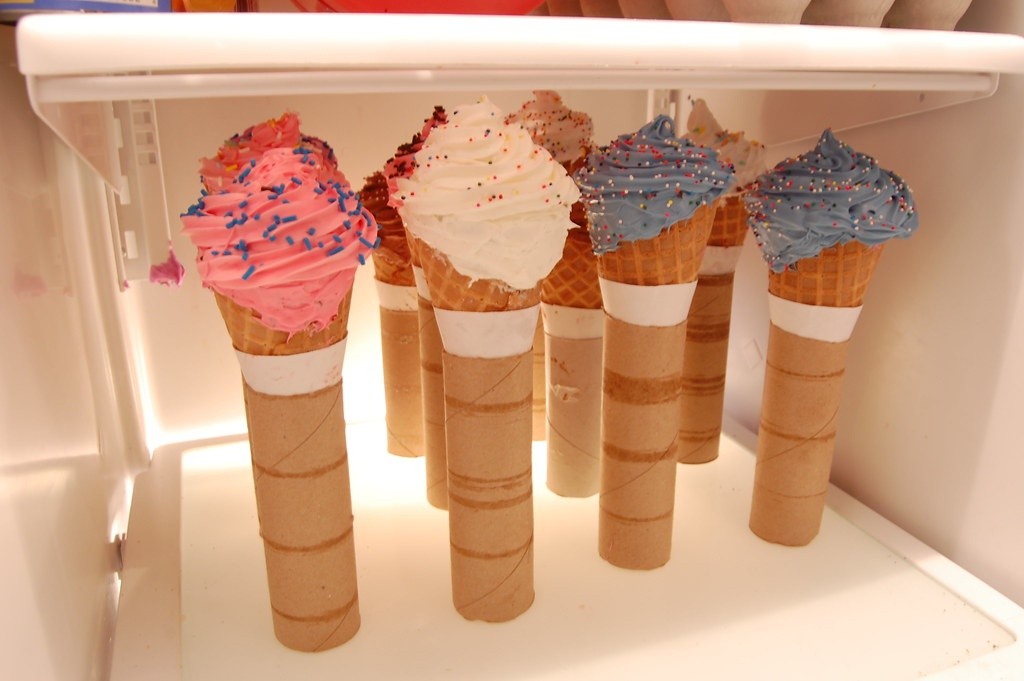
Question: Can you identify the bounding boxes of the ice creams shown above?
[178,89,919,655]
